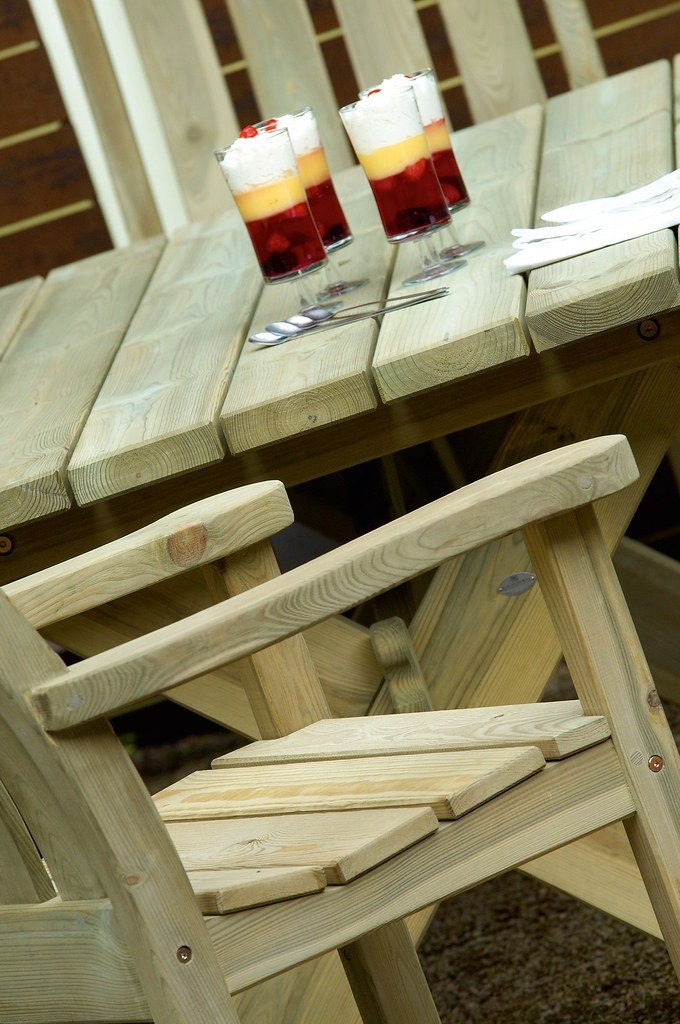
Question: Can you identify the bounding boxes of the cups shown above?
[216,126,331,286]
[337,85,452,246]
[245,107,358,256]
[359,66,472,215]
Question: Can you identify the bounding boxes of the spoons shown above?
[248,289,451,348]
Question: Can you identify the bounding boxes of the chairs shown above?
[0,434,679,1022]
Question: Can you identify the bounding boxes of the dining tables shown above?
[0,53,679,1023]
